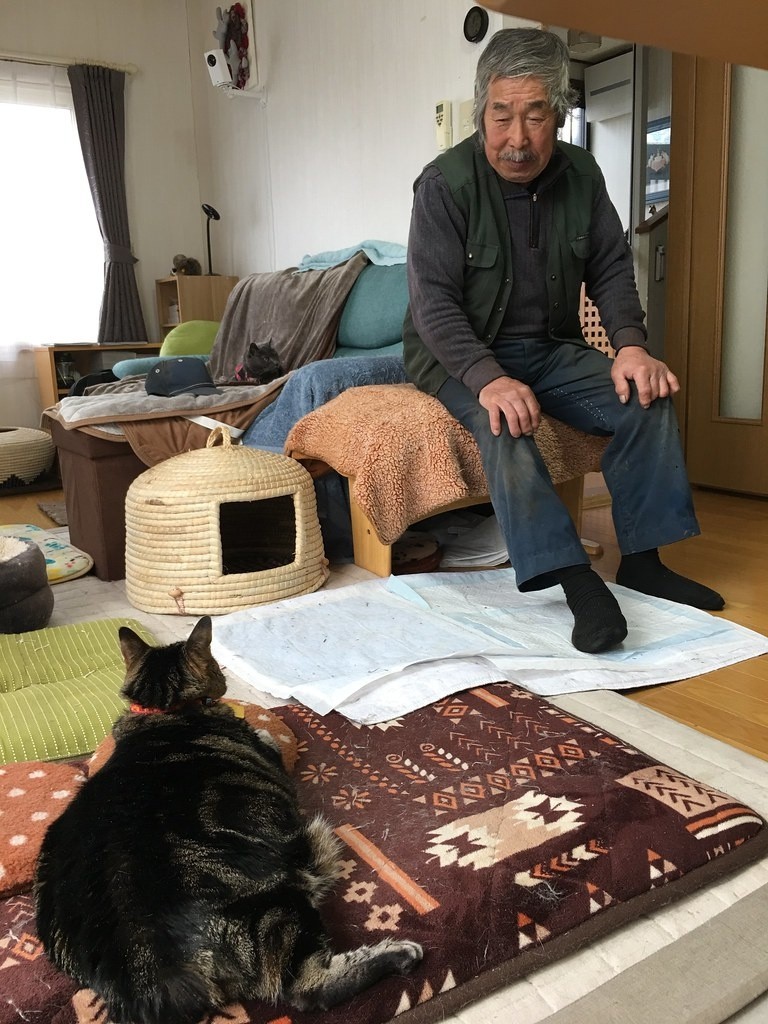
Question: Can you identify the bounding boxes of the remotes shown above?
[436,100,452,150]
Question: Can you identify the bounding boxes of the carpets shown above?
[37,500,68,526]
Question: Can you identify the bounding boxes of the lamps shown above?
[202,203,222,276]
[566,28,602,53]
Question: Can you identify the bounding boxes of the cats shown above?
[30,613,427,1024]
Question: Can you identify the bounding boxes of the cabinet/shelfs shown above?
[31,342,166,435]
[153,275,241,342]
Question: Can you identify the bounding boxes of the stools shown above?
[48,415,154,583]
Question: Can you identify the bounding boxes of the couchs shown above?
[83,238,414,564]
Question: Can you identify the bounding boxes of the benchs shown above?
[280,382,615,580]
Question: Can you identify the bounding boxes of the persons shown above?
[401,28,724,655]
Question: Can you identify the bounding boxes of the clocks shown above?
[463,6,488,43]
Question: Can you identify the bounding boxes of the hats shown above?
[145,357,223,396]
